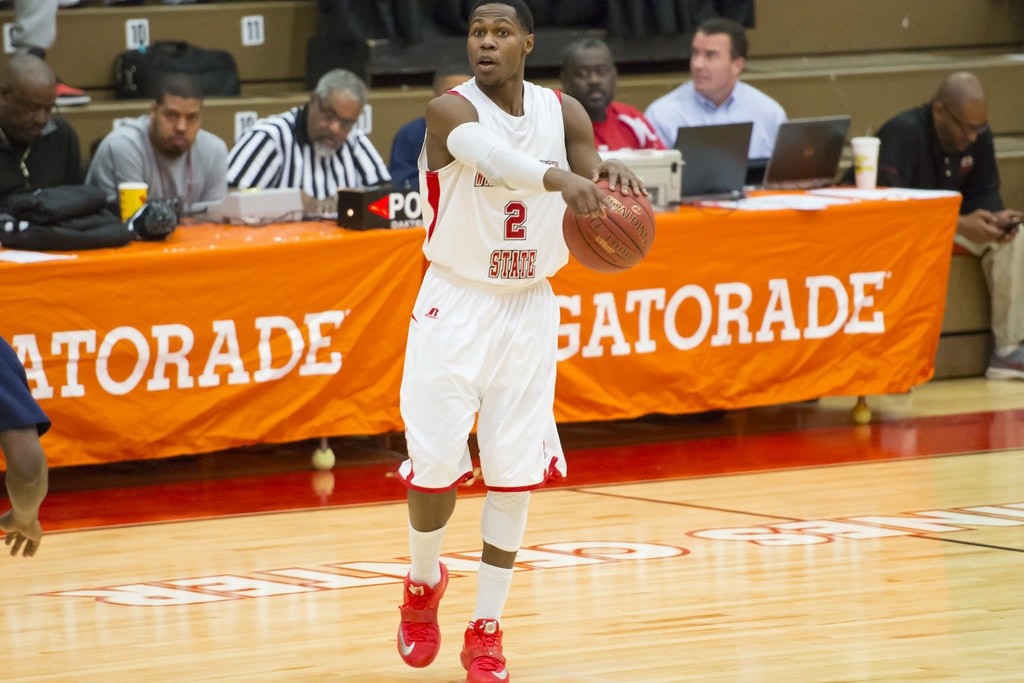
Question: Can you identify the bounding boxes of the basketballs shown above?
[561,178,656,273]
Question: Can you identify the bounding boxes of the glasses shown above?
[19,103,61,119]
[318,103,357,128]
[945,106,990,136]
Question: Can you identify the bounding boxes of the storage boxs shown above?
[597,147,686,210]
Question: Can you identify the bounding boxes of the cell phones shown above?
[996,218,1022,233]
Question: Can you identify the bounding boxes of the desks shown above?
[0,185,962,474]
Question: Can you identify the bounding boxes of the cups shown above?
[849,136,881,190]
[117,182,149,224]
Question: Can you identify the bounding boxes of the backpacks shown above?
[0,185,129,252]
[111,39,240,102]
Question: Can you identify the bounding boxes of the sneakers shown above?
[984,343,1024,380]
[398,560,448,669]
[460,618,510,683]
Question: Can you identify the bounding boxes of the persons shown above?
[0,55,82,218]
[837,70,1024,381]
[84,72,230,223]
[553,36,666,155]
[400,3,646,683]
[645,18,788,160]
[0,336,51,559]
[10,0,91,107]
[388,60,475,189]
[227,68,393,215]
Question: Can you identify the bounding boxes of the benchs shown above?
[51,0,1024,381]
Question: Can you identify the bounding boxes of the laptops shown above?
[674,122,753,201]
[757,117,851,191]
